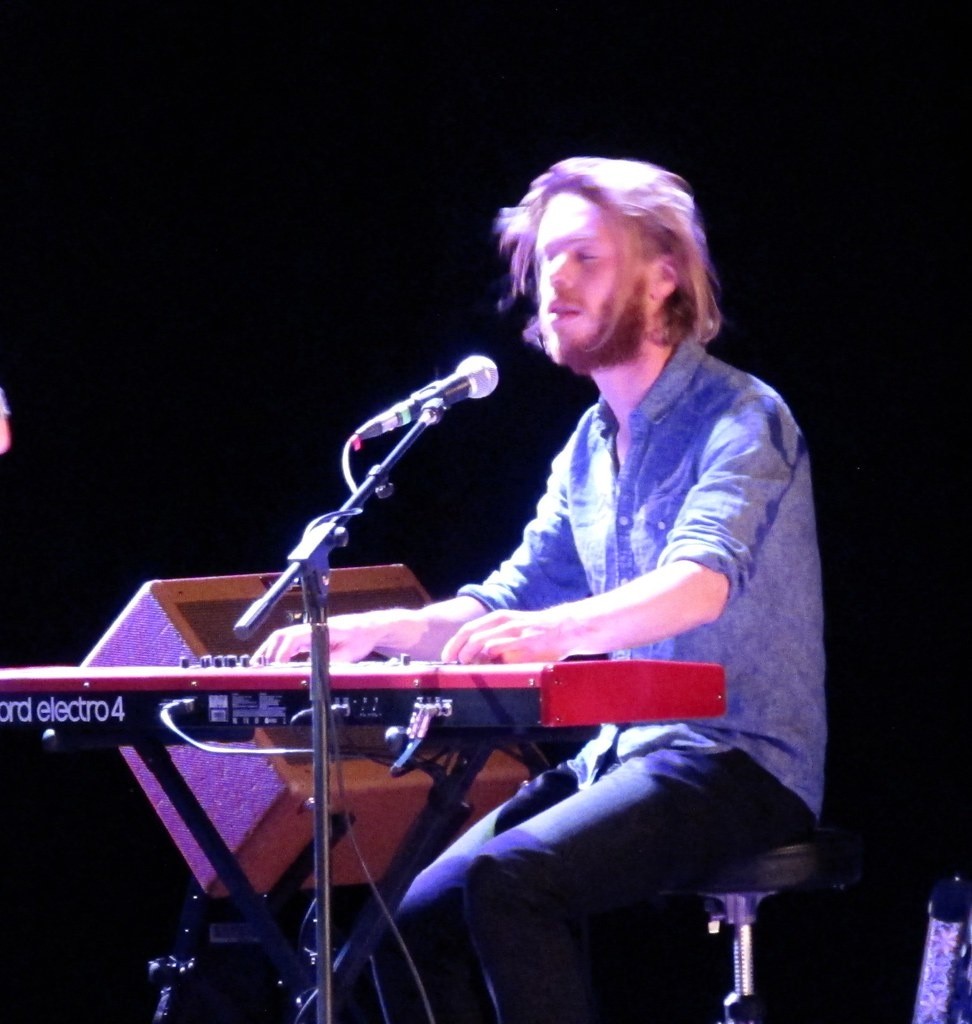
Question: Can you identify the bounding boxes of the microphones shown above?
[354,354,499,440]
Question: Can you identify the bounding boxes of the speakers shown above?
[77,564,545,903]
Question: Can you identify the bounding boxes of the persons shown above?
[247,156,829,1024]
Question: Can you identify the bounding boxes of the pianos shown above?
[1,652,728,1024]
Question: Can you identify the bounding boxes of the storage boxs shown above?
[81,561,532,893]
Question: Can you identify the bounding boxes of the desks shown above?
[41,723,511,1024]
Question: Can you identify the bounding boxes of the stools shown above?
[698,840,827,1024]
